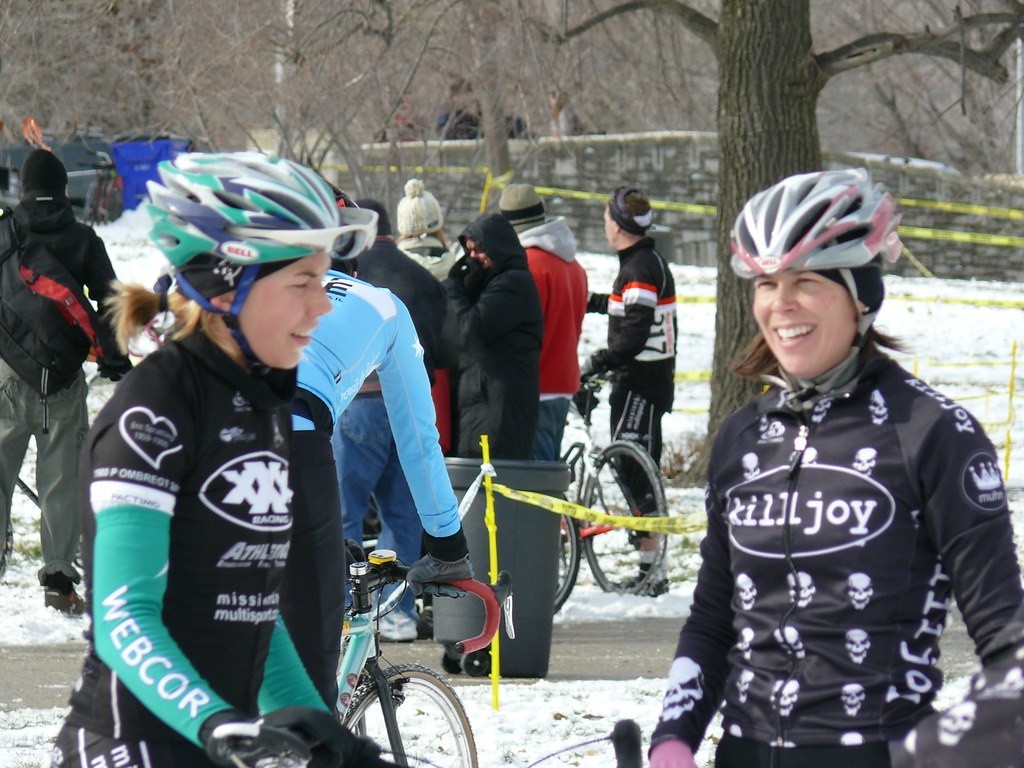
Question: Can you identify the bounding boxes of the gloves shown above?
[648,739,699,768]
[406,553,473,598]
[199,707,314,768]
[97,355,134,382]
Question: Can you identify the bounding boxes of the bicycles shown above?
[553,368,670,617]
[288,547,515,768]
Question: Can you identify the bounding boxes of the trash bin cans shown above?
[431,454,572,680]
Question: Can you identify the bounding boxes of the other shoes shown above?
[370,605,418,642]
[621,576,668,597]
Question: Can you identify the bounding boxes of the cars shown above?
[0,130,124,225]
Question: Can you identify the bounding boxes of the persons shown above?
[645,166,1024,768]
[345,177,587,642]
[437,78,484,140]
[289,243,469,707]
[544,86,581,137]
[584,186,677,598]
[51,150,332,768]
[0,148,133,614]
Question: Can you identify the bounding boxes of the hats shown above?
[397,178,442,235]
[20,150,68,201]
[499,183,546,232]
[354,199,391,235]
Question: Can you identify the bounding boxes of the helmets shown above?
[730,168,903,278]
[144,151,378,299]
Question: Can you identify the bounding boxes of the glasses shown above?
[224,206,380,260]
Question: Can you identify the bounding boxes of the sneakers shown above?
[45,588,85,614]
